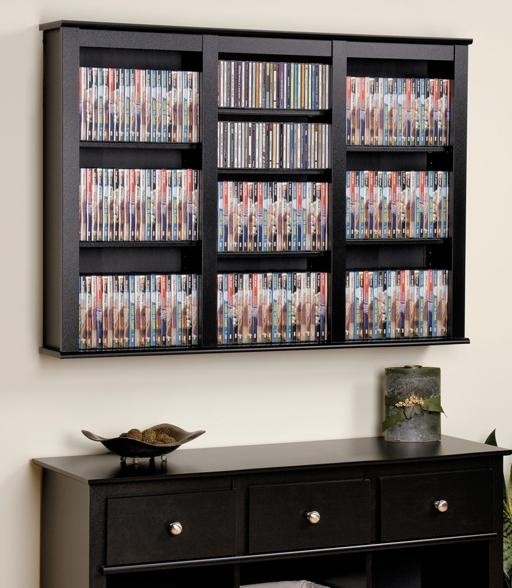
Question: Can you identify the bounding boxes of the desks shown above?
[32,437,511,586]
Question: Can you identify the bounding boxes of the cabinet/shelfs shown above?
[40,21,473,360]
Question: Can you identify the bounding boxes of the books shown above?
[217,273,328,343]
[346,76,449,146]
[80,275,199,348]
[79,66,201,144]
[345,271,450,339]
[216,61,330,170]
[345,170,449,238]
[80,168,202,241]
[216,181,329,252]
